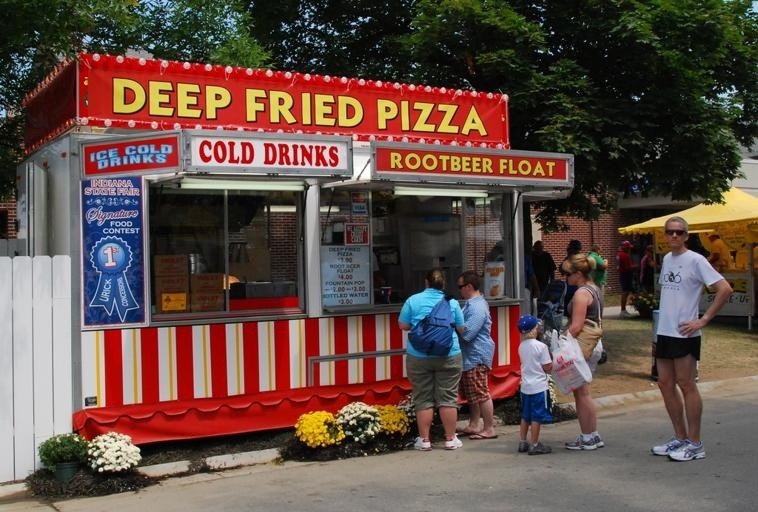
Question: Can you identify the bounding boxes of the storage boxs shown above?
[153,253,227,316]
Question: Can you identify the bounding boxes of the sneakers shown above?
[413,435,432,451]
[564,433,597,450]
[527,440,552,455]
[667,438,706,461]
[619,309,633,319]
[594,435,605,448]
[444,433,463,450]
[650,437,688,456]
[518,440,529,452]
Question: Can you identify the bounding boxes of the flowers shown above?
[38,432,87,468]
[629,291,659,309]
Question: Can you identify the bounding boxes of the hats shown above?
[623,239,635,249]
[705,230,720,237]
[517,313,543,335]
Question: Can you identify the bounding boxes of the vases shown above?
[637,307,650,320]
[50,462,78,483]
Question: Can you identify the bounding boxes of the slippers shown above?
[468,432,498,440]
[455,428,476,437]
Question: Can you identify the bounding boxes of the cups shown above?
[488,279,502,297]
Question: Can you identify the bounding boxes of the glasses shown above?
[664,229,688,236]
[457,282,471,289]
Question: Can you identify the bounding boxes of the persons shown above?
[532,240,556,292]
[559,240,582,316]
[562,253,605,452]
[516,314,553,456]
[459,269,498,440]
[707,229,731,274]
[398,266,465,451]
[639,244,661,293]
[586,245,608,319]
[648,216,734,462]
[615,240,640,319]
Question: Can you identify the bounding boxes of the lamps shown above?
[175,180,308,192]
[391,187,494,199]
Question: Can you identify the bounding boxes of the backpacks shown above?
[406,293,457,357]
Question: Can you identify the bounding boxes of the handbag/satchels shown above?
[562,286,603,360]
